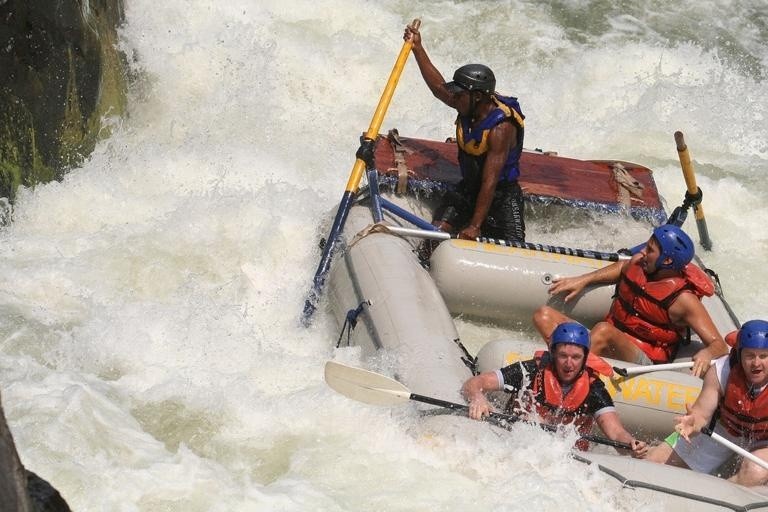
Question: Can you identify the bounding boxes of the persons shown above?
[459,321,650,460]
[642,319,768,487]
[531,225,730,379]
[402,22,525,269]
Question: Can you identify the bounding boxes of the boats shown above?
[332,130,765,512]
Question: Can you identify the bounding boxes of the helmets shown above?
[453,64,497,98]
[735,319,768,363]
[549,320,592,361]
[654,224,695,271]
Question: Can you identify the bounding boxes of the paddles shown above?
[323,359,639,450]
